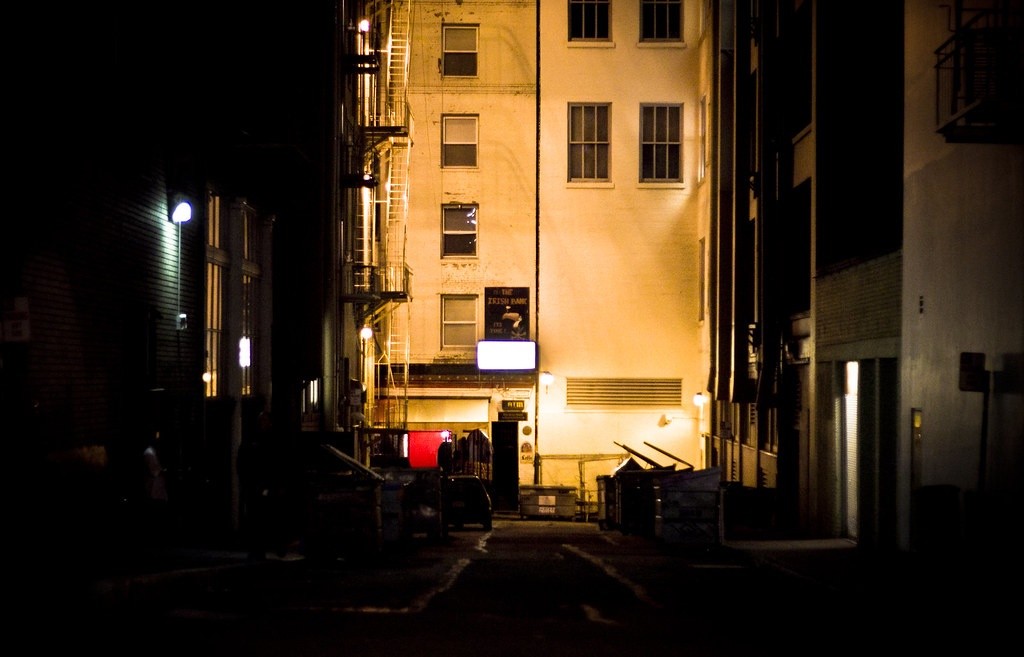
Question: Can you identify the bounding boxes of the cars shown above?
[443,475,493,531]
